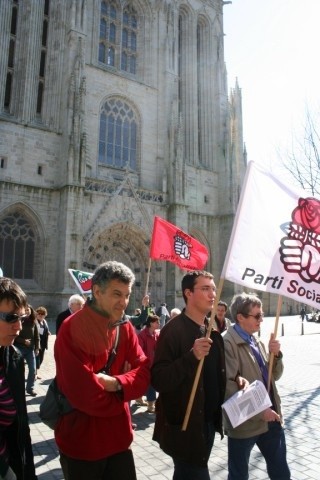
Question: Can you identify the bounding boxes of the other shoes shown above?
[131,421,138,428]
[134,399,149,406]
[26,389,38,396]
[35,374,43,381]
[147,406,155,413]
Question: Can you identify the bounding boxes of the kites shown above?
[218,159,320,309]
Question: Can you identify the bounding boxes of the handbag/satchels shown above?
[40,378,76,430]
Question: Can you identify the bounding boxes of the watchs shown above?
[115,378,121,391]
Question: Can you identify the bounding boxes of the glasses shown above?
[244,313,262,319]
[0,312,27,324]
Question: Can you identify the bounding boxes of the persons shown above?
[0,277,38,480]
[220,293,292,480]
[152,271,227,480]
[54,261,151,480]
[54,293,231,429]
[12,303,49,397]
[300,308,305,321]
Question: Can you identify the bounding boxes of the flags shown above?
[150,215,209,271]
[68,269,95,294]
